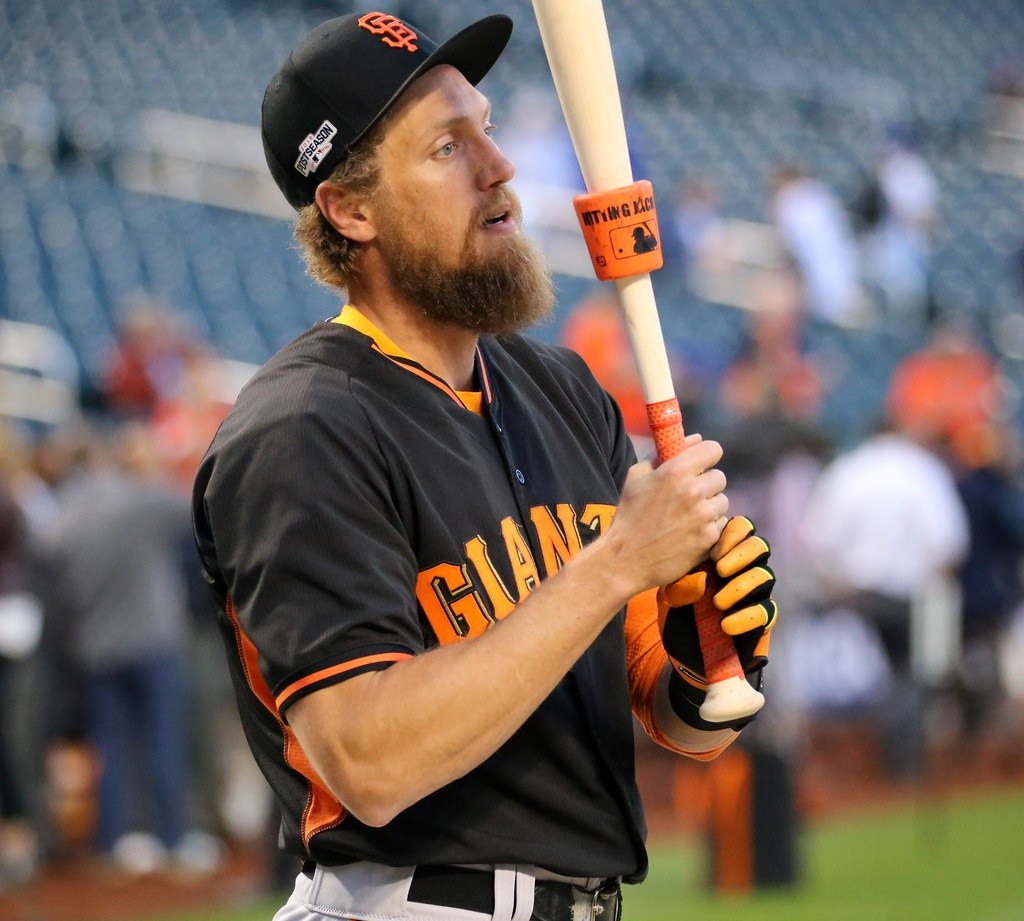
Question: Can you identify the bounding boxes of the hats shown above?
[262,11,513,214]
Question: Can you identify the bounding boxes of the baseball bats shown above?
[531,1,768,726]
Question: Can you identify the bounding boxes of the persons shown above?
[191,13,780,920]
[1,125,1024,891]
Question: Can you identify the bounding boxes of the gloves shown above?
[657,516,777,731]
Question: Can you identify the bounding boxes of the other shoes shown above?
[114,833,169,876]
[171,834,223,879]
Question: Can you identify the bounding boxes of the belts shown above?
[301,859,623,921]
